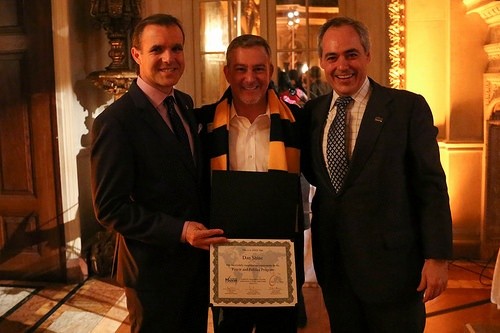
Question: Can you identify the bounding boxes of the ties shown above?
[326,96,355,192]
[162,96,194,168]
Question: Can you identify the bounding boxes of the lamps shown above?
[88,0,139,71]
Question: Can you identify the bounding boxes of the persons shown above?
[91,14,227,332]
[299,17,452,333]
[277,62,331,109]
[195,34,304,333]
